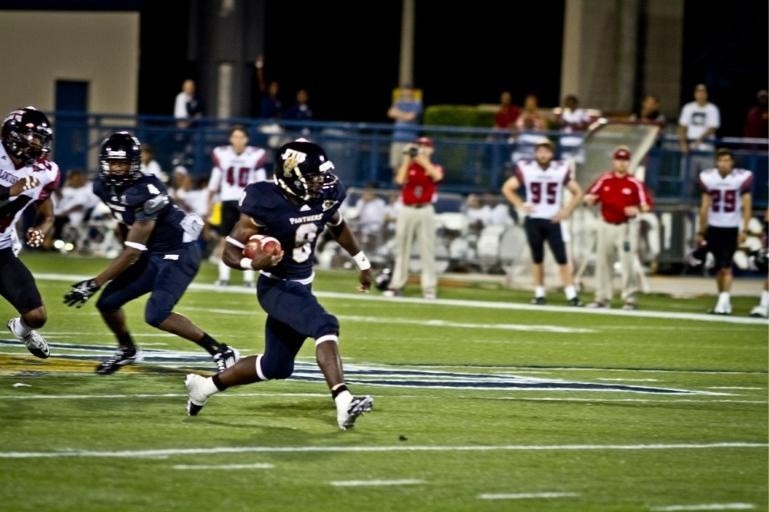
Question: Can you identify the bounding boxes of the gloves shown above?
[61,278,102,309]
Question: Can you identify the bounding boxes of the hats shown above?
[534,137,555,150]
[415,135,433,150]
[609,144,633,162]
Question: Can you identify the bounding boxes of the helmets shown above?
[1,105,56,167]
[96,131,143,188]
[273,141,341,208]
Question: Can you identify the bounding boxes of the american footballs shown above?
[244,235,282,260]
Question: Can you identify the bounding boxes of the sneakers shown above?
[383,287,401,297]
[212,344,240,374]
[337,394,374,432]
[749,307,768,318]
[568,296,584,307]
[587,300,611,309]
[424,287,437,303]
[94,344,143,376]
[715,291,733,316]
[184,372,211,417]
[7,316,51,359]
[531,297,546,305]
[623,301,637,311]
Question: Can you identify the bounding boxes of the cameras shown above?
[404,148,419,158]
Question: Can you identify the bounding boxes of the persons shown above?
[185,142,374,431]
[0,106,60,357]
[63,130,239,377]
